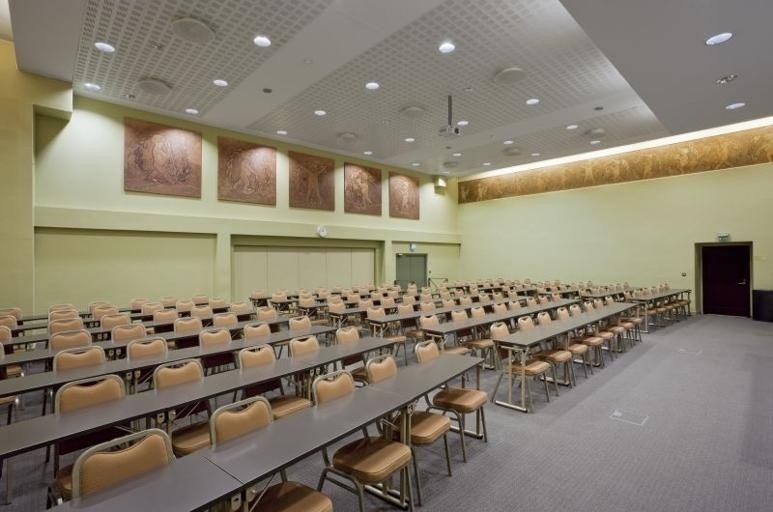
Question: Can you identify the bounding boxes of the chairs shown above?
[555,307,605,376]
[46,316,85,336]
[421,286,432,293]
[477,293,491,303]
[418,314,472,382]
[208,295,229,307]
[251,289,267,297]
[2,314,18,329]
[313,368,418,510]
[335,325,368,388]
[402,294,416,304]
[452,288,465,297]
[380,296,396,306]
[604,295,644,346]
[228,300,250,313]
[371,291,384,299]
[190,304,214,317]
[365,354,453,491]
[333,282,346,292]
[442,298,455,321]
[173,316,204,349]
[152,358,211,457]
[358,297,374,308]
[153,308,180,322]
[419,293,433,301]
[508,299,523,310]
[100,311,133,332]
[313,285,324,292]
[110,321,148,341]
[255,306,291,359]
[130,296,149,308]
[419,301,437,310]
[47,301,76,314]
[470,305,486,317]
[351,280,360,290]
[469,288,479,295]
[325,293,347,310]
[489,320,552,414]
[569,303,615,370]
[125,335,170,361]
[340,286,354,297]
[396,303,423,354]
[347,293,361,302]
[326,294,343,304]
[0,346,22,378]
[272,292,288,299]
[90,305,120,316]
[242,323,272,339]
[141,301,165,313]
[298,297,316,306]
[0,393,17,424]
[516,316,573,396]
[491,291,503,301]
[458,295,473,305]
[43,373,137,512]
[192,293,210,305]
[407,288,417,294]
[299,287,314,306]
[41,327,95,418]
[90,297,114,308]
[451,308,496,370]
[237,342,315,422]
[287,315,313,331]
[174,297,197,309]
[537,295,549,304]
[367,306,393,352]
[289,335,327,403]
[438,291,452,299]
[69,426,175,502]
[318,288,331,298]
[207,395,336,512]
[526,296,537,307]
[506,290,518,297]
[198,325,233,368]
[387,290,399,297]
[537,310,590,388]
[492,301,519,334]
[0,321,14,343]
[47,307,79,320]
[437,282,448,290]
[551,292,562,301]
[160,294,179,307]
[357,284,369,294]
[42,344,110,465]
[1,306,21,317]
[211,311,241,341]
[582,300,624,360]
[414,339,488,463]
[592,298,635,353]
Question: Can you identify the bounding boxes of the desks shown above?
[0,310,257,347]
[419,298,582,352]
[445,282,507,290]
[249,289,376,310]
[20,302,208,326]
[538,287,600,297]
[43,352,487,512]
[328,292,496,327]
[490,301,639,415]
[297,290,455,322]
[478,283,543,294]
[623,288,692,335]
[3,314,291,373]
[514,285,566,298]
[270,290,408,315]
[365,296,533,362]
[9,305,229,336]
[579,287,637,299]
[0,336,408,460]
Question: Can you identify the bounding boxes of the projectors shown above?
[439,125,460,139]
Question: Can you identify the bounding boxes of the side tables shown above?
[0,324,338,400]
